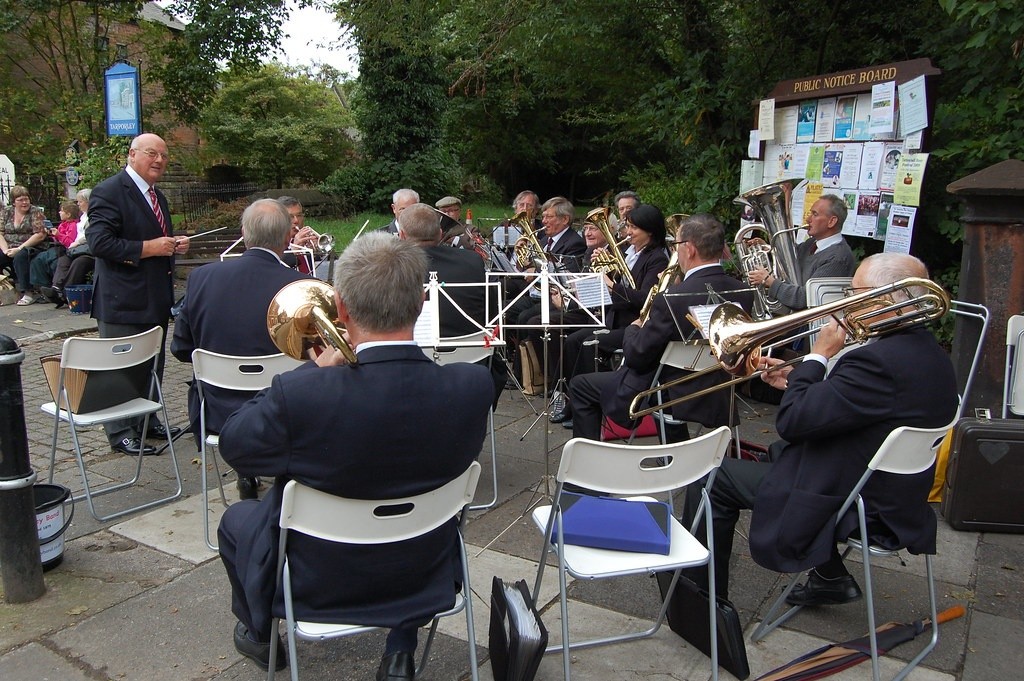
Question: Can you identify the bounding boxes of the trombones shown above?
[628,276,953,421]
[266,277,360,368]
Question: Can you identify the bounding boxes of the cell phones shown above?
[43,220,55,233]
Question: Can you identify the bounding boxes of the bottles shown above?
[554,390,565,414]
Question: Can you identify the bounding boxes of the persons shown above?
[0,185,93,310]
[372,189,506,412]
[217,232,496,681]
[492,190,754,498]
[277,196,319,276]
[170,198,325,500]
[748,194,854,406]
[682,253,958,606]
[85,133,189,456]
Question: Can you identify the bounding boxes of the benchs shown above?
[3,222,95,309]
[174,229,243,267]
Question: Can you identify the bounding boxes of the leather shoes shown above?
[505,380,522,389]
[233,620,287,672]
[539,389,554,398]
[549,412,573,428]
[781,566,863,605]
[138,424,181,440]
[111,437,157,456]
[376,651,415,681]
[237,476,261,500]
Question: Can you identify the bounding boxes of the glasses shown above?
[441,209,461,213]
[842,286,878,297]
[669,240,689,252]
[133,148,171,162]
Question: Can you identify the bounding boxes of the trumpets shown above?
[294,224,337,255]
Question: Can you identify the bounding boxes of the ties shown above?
[547,238,554,253]
[809,242,818,256]
[148,187,168,238]
[291,239,309,274]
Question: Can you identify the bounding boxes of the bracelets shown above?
[18,247,21,251]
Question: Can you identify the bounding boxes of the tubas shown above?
[435,208,465,245]
[585,205,636,292]
[507,209,548,292]
[637,213,693,327]
[734,175,809,319]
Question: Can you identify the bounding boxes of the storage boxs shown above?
[39,353,140,414]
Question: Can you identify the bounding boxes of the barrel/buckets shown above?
[32,482,75,572]
[64,284,94,315]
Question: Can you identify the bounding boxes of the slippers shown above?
[16,292,40,305]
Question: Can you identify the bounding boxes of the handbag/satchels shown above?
[519,339,555,395]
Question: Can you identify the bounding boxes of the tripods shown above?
[477,218,607,558]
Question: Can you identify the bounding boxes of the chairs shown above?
[268,461,481,681]
[531,425,732,680]
[626,341,741,520]
[40,325,182,521]
[595,344,625,372]
[1002,314,1024,419]
[751,394,963,681]
[806,277,989,437]
[192,348,306,551]
[419,329,506,510]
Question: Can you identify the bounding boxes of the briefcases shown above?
[939,416,1023,535]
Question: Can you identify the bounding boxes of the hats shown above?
[435,196,462,207]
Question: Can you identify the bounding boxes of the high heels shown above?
[40,286,68,309]
